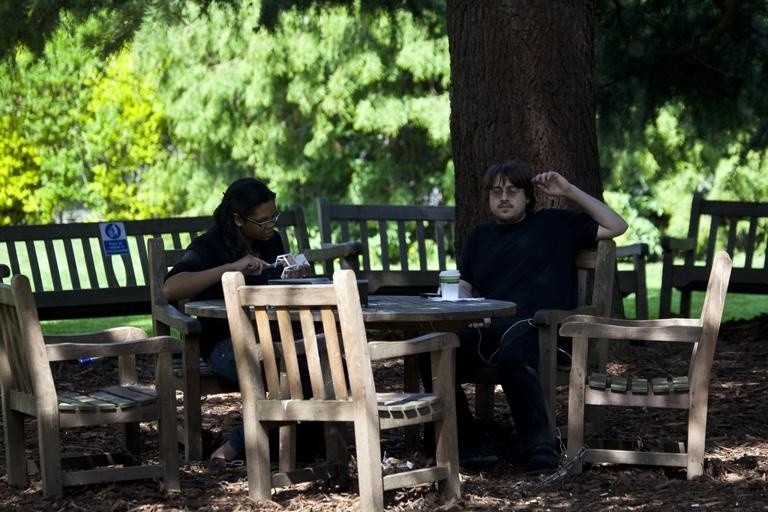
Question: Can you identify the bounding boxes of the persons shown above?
[161,178,327,462]
[420,160,629,476]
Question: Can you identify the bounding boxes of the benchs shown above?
[0,204,369,340]
[655,188,768,321]
[312,195,650,320]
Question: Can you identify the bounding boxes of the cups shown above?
[437,270,461,301]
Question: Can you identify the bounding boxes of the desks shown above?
[176,289,521,474]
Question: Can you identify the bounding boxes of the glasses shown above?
[237,209,280,228]
[491,189,518,196]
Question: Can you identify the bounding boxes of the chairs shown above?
[0,273,189,501]
[215,266,465,512]
[556,246,733,485]
[144,232,241,467]
[397,234,618,442]
